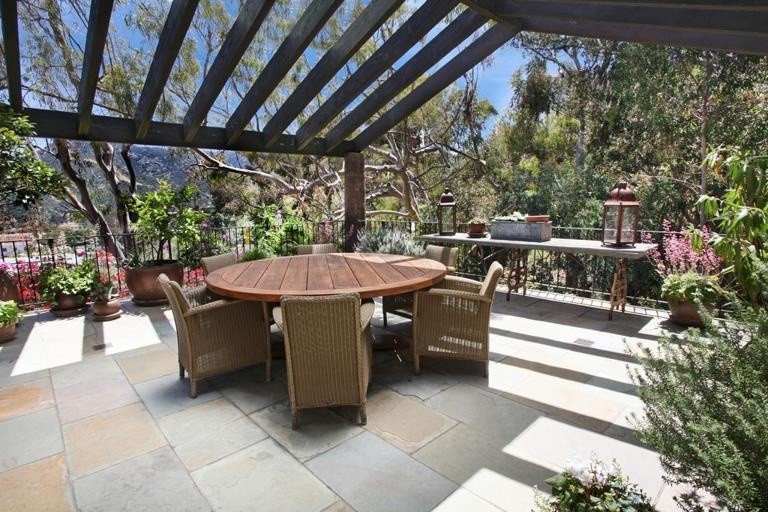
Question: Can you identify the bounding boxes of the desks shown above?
[413,233,658,322]
[204,252,447,363]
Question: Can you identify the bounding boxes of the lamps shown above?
[436,188,456,236]
[601,182,640,248]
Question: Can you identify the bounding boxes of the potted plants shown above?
[237,248,277,263]
[490,211,553,242]
[121,177,211,307]
[467,217,487,238]
[42,257,97,317]
[89,279,123,321]
[0,300,19,344]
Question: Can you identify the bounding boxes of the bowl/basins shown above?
[491,220,552,242]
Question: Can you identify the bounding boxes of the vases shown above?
[665,298,714,328]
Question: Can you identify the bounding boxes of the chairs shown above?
[295,244,333,255]
[412,260,503,379]
[382,244,458,338]
[273,292,375,431]
[199,252,276,327]
[155,274,271,399]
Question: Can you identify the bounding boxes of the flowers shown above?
[640,220,729,304]
[352,226,426,257]
